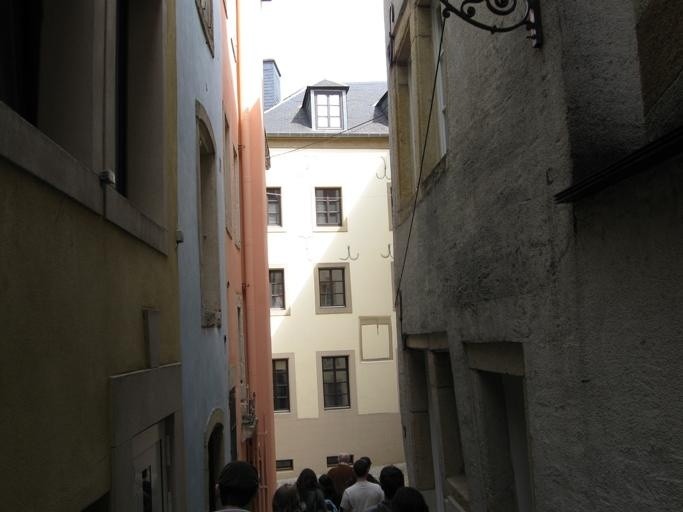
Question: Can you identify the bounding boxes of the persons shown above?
[271,451,430,512]
[210,459,258,512]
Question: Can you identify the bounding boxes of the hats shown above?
[217,460,258,490]
